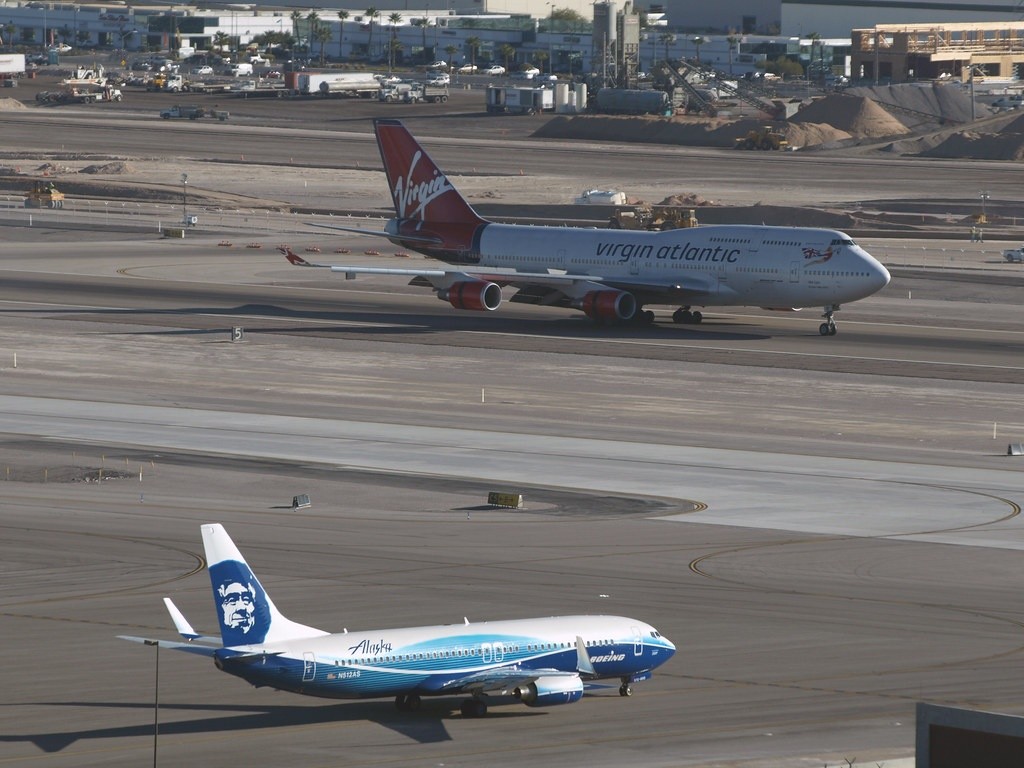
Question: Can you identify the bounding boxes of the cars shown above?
[483,64,506,77]
[520,66,541,77]
[456,63,478,74]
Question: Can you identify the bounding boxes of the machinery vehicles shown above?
[606,202,699,231]
[23,179,64,209]
[733,125,788,150]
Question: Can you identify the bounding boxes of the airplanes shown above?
[273,118,892,336]
[113,522,678,720]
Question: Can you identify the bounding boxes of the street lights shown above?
[546,1,558,76]
[806,58,822,97]
[979,193,991,215]
[141,638,161,768]
[180,171,190,225]
[121,30,138,75]
[39,5,47,47]
[966,62,985,124]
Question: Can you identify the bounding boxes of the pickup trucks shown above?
[991,96,1024,108]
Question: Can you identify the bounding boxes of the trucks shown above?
[0,40,451,106]
[1003,246,1024,263]
[159,103,205,121]
[573,188,628,205]
